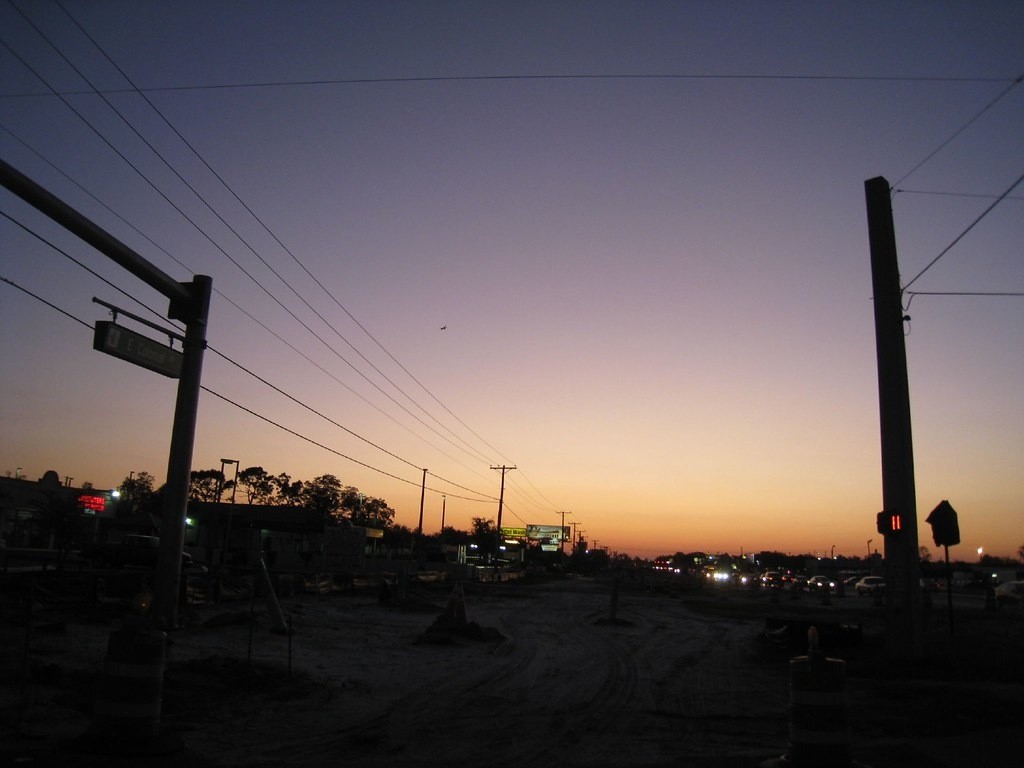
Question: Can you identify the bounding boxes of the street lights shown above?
[867,540,873,575]
[127,471,136,503]
[831,545,835,560]
[440,495,446,534]
[214,457,241,605]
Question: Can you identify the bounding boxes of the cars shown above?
[700,567,864,591]
[855,575,893,597]
[994,582,1024,603]
[81,535,192,569]
